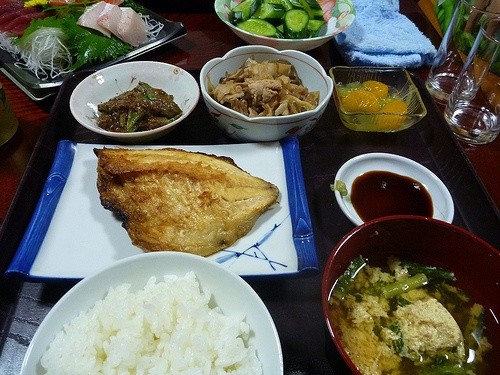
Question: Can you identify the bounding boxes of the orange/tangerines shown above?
[341,80,408,131]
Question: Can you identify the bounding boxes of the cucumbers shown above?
[228,0,326,39]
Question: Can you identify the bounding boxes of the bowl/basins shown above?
[17,251,285,375]
[69,61,200,140]
[215,0,356,51]
[329,65,427,131]
[201,44,333,142]
[323,216,500,375]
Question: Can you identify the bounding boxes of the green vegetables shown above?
[16,0,149,72]
[333,254,491,375]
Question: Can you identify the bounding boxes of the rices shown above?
[40,269,264,375]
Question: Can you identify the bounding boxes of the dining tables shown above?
[0,0,500,375]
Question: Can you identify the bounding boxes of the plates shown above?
[0,1,188,102]
[5,140,321,281]
[333,153,454,225]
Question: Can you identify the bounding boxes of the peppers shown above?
[125,80,183,131]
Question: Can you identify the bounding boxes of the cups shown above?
[443,16,500,147]
[425,0,500,106]
[0,82,18,147]
